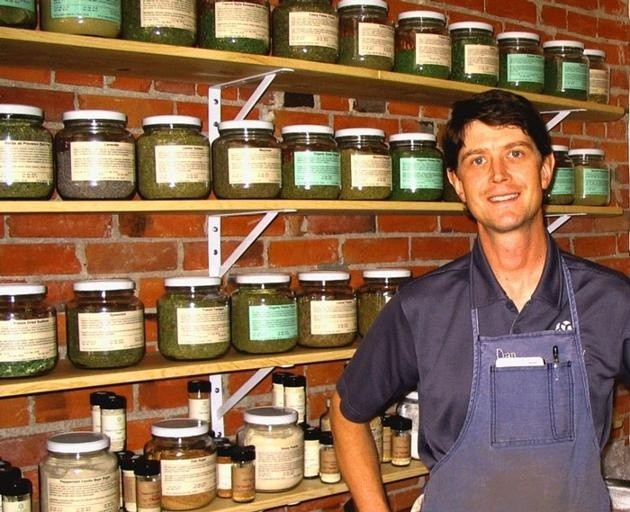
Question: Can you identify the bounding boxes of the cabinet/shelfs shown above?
[1,20,624,512]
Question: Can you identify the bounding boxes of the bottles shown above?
[389,132,443,201]
[394,9,451,81]
[303,426,320,479]
[320,432,341,484]
[450,21,498,86]
[297,271,358,349]
[391,418,412,468]
[41,0,122,37]
[217,444,240,498]
[188,381,211,423]
[0,104,55,200]
[235,407,305,493]
[272,0,341,63]
[0,466,22,512]
[156,275,231,362]
[38,431,120,512]
[383,414,390,463]
[0,459,10,467]
[0,0,39,30]
[212,120,283,201]
[136,460,160,512]
[569,148,611,206]
[499,31,545,93]
[396,391,419,461]
[232,446,259,503]
[335,126,393,200]
[358,268,412,346]
[282,124,342,199]
[125,0,197,46]
[115,450,135,508]
[231,271,298,355]
[55,109,138,198]
[583,47,610,105]
[368,416,385,464]
[320,398,332,431]
[336,0,394,71]
[544,144,575,204]
[101,395,126,453]
[542,39,590,101]
[135,114,211,200]
[272,371,294,409]
[2,477,33,512]
[214,437,229,443]
[200,1,270,56]
[283,375,307,424]
[1,284,58,379]
[65,277,146,369]
[121,454,145,512]
[89,391,116,433]
[144,419,217,511]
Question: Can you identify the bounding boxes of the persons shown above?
[330,88,630,512]
